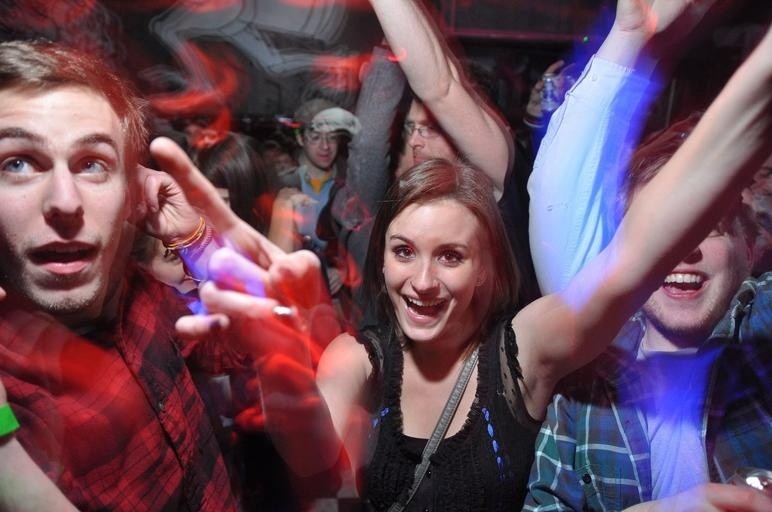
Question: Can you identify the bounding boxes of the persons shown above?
[283,97,355,301]
[738,197,772,279]
[526,0,714,301]
[0,390,81,512]
[131,233,197,302]
[148,29,771,512]
[0,1,152,88]
[522,112,772,512]
[524,58,582,155]
[740,152,771,200]
[193,130,317,251]
[330,36,409,298]
[1,40,249,512]
[367,2,521,330]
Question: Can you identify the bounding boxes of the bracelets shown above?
[161,214,208,252]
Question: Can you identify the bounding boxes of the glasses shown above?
[303,130,339,146]
[404,123,445,139]
